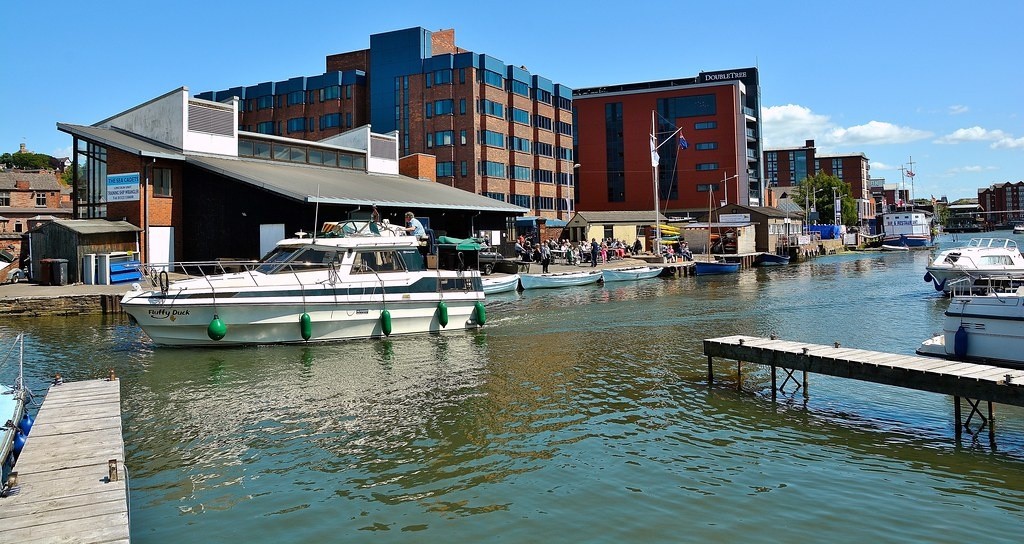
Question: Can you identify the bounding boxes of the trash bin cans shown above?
[39,258,69,286]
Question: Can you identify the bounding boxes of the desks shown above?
[580,250,621,262]
[550,250,568,267]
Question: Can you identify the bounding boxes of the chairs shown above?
[665,255,677,263]
[676,250,687,262]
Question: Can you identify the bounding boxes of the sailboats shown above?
[625,108,684,265]
[749,197,791,268]
[694,184,743,277]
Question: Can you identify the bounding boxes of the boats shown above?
[647,223,682,249]
[914,274,1024,371]
[0,330,36,472]
[923,236,1024,298]
[601,265,665,284]
[880,242,912,252]
[119,176,487,350]
[1013,223,1024,235]
[881,233,930,248]
[517,270,603,291]
[480,273,521,295]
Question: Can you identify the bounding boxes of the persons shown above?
[930,224,943,243]
[663,243,674,260]
[974,275,984,295]
[485,235,490,246]
[515,231,643,274]
[401,212,428,269]
[675,236,693,261]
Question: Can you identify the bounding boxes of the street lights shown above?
[567,160,581,222]
[811,187,823,227]
[720,170,738,207]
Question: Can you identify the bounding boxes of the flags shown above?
[679,131,687,148]
[907,171,915,176]
